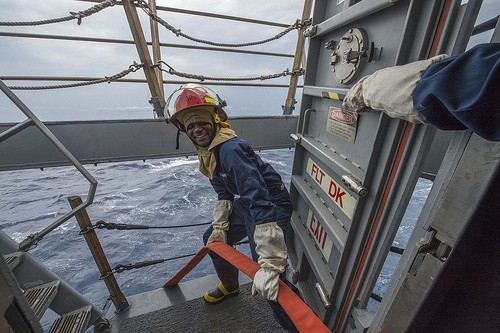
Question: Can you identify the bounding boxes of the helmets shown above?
[163,82,228,126]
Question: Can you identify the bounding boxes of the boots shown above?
[203,258,239,304]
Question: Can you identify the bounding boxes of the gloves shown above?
[342,53,450,126]
[250,220,290,302]
[206,198,233,260]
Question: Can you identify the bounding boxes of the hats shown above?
[183,108,219,130]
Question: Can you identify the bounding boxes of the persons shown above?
[341,42,500,141]
[163,83,319,333]
